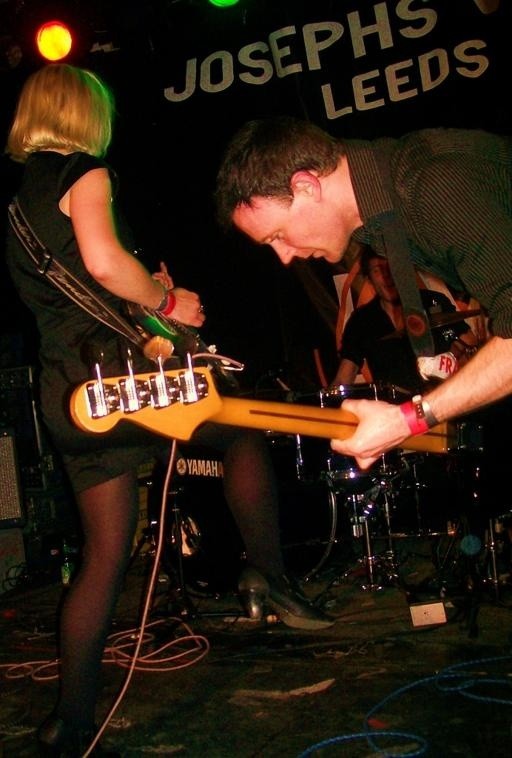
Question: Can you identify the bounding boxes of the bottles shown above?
[58,535,75,586]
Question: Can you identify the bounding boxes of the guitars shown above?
[68,352,512,520]
[124,259,239,393]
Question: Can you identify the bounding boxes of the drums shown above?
[148,422,375,602]
[295,383,410,490]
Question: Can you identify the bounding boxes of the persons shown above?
[1,62,337,758]
[326,246,478,403]
[213,116,512,472]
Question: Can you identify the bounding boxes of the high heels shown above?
[37,709,122,758]
[236,563,335,631]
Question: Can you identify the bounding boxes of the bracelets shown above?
[400,394,439,436]
[156,285,176,315]
